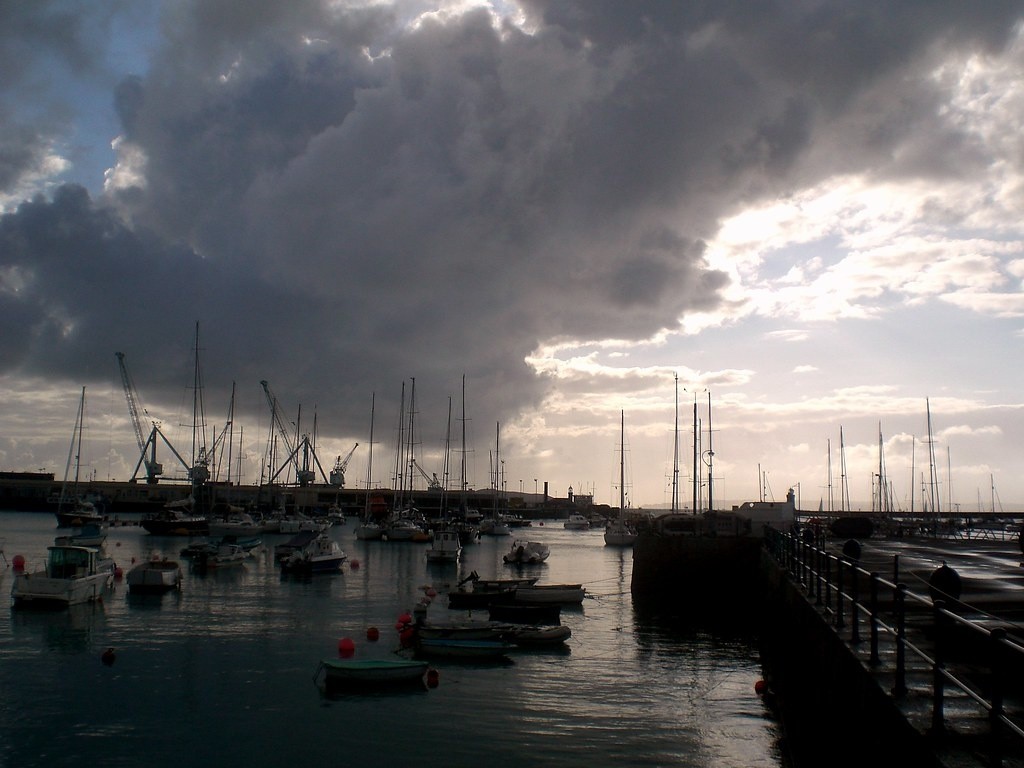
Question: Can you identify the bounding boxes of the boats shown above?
[311,656,433,694]
[124,558,185,588]
[9,543,119,608]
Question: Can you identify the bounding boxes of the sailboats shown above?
[48,316,1024,648]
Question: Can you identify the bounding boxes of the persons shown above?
[221,504,231,524]
[414,597,428,625]
